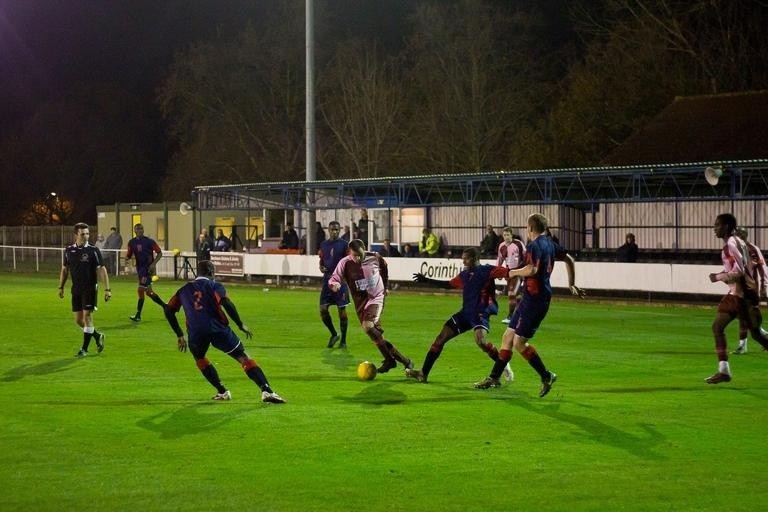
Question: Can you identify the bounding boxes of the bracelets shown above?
[104,288,111,291]
[58,286,64,289]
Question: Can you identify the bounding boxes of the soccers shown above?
[358,360,376,381]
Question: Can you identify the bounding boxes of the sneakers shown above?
[328,333,340,347]
[75,349,88,356]
[378,358,397,373]
[473,376,500,388]
[340,343,346,349]
[405,359,413,368]
[130,316,141,321]
[729,346,747,354]
[262,391,286,403]
[504,363,514,382]
[704,372,731,384]
[501,318,511,324]
[97,334,104,352]
[212,390,231,400]
[540,371,557,397]
[406,369,427,382]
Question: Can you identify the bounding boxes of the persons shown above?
[57,222,112,356]
[319,222,351,350]
[476,213,586,396]
[730,227,767,354]
[94,233,110,273]
[126,224,166,321]
[615,233,639,262]
[328,239,414,373]
[495,226,525,324]
[705,213,768,383]
[104,227,122,276]
[164,260,286,403]
[405,247,526,383]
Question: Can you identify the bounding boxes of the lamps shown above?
[178,202,197,216]
[704,166,724,186]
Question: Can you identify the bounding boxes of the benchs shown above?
[441,247,723,265]
[262,248,303,255]
